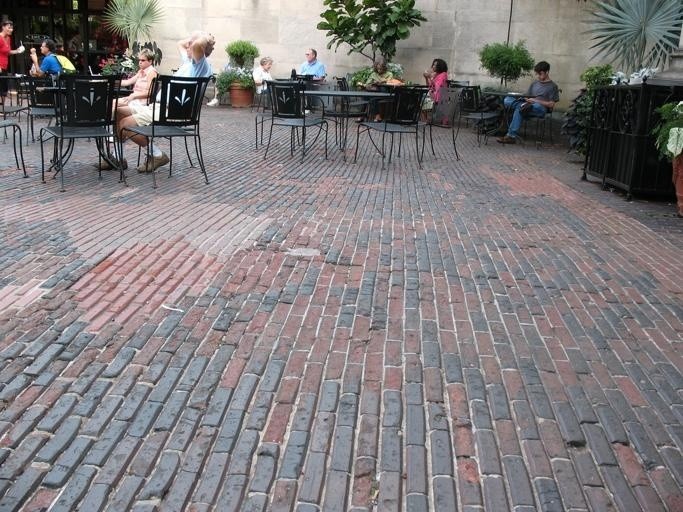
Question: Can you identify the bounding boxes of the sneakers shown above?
[374,114,382,122]
[95,154,127,170]
[520,102,533,112]
[355,116,368,122]
[137,151,169,172]
[497,136,516,144]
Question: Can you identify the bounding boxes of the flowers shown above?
[215,68,256,96]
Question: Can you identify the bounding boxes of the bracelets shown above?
[429,77,433,81]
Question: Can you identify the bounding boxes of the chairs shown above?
[0,57,216,192]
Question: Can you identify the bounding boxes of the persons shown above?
[297,49,327,118]
[497,61,560,144]
[0,21,27,119]
[353,56,394,123]
[31,40,77,118]
[206,57,256,108]
[28,34,85,66]
[92,31,217,174]
[420,59,451,126]
[253,57,275,110]
[109,48,160,162]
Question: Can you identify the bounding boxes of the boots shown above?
[418,109,432,125]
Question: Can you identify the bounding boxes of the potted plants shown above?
[648,99,683,219]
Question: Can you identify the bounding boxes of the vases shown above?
[228,80,253,108]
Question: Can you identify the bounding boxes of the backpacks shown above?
[53,55,77,76]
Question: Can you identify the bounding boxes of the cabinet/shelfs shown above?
[579,75,682,203]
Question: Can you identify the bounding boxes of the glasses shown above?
[138,59,148,62]
